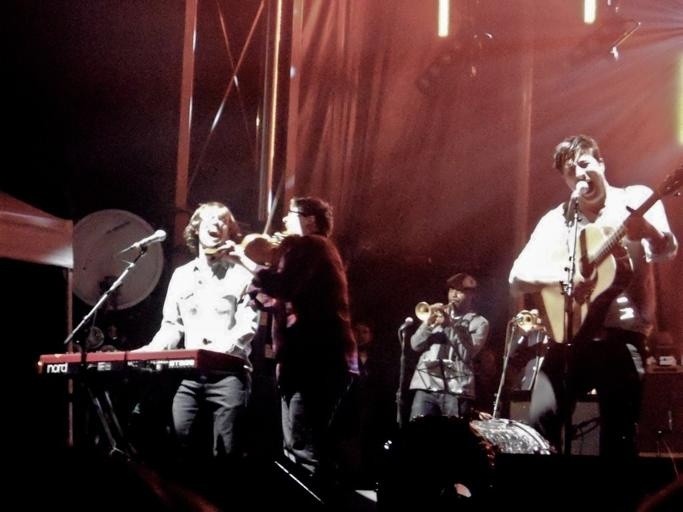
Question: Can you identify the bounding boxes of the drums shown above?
[378,416,555,511]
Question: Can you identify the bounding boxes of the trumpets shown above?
[518,310,544,333]
[415,302,456,321]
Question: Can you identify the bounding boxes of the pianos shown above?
[37,350,246,378]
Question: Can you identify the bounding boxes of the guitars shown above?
[534,164,682,343]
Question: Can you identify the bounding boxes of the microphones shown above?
[570,179,590,206]
[133,228,167,250]
[399,316,414,333]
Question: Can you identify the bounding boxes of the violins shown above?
[230,233,295,272]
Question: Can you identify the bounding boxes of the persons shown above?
[101,202,261,460]
[508,134,679,511]
[368,416,500,512]
[249,196,353,511]
[352,323,371,385]
[408,272,490,423]
